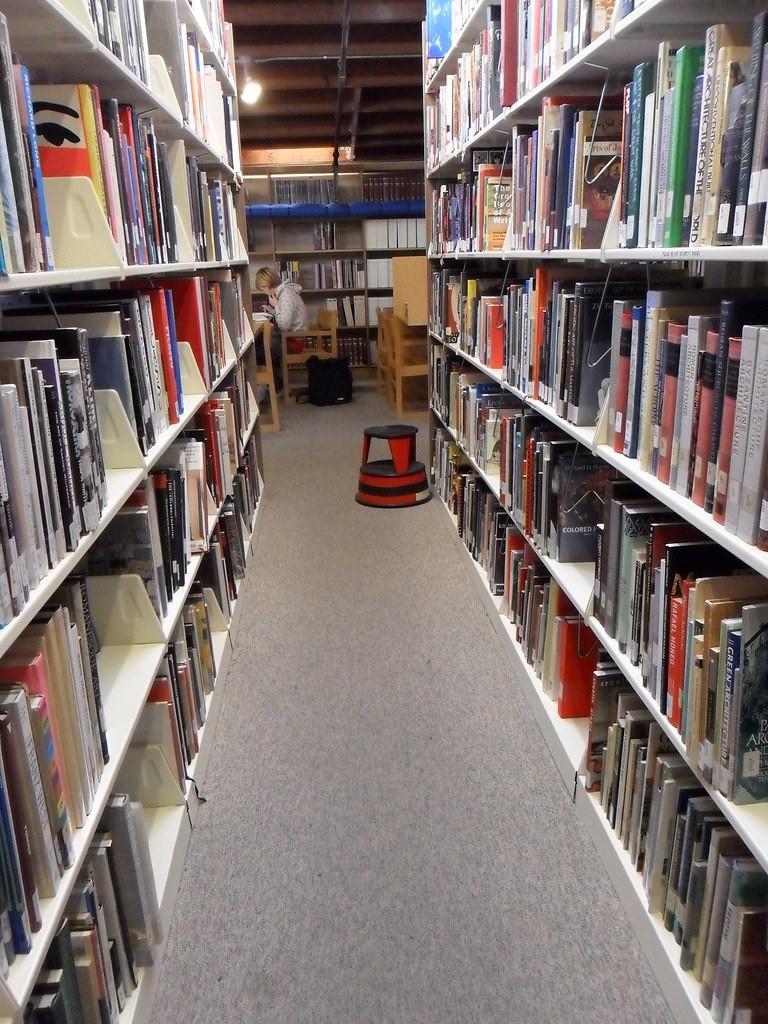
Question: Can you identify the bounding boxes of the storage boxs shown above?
[391,257,428,326]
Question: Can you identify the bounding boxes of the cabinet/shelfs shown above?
[243,162,427,370]
[418,0,768,1023]
[0,0,263,1024]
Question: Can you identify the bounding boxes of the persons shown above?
[255,267,307,397]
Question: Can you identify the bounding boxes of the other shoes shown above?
[276,386,291,397]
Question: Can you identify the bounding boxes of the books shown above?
[271,173,427,369]
[427,0,768,1024]
[1,0,265,1024]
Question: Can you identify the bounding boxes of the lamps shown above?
[237,56,263,105]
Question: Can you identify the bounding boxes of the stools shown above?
[354,424,433,508]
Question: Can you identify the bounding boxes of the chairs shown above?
[375,307,429,419]
[281,307,338,404]
[256,322,282,432]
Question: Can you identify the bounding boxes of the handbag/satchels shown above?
[296,355,353,407]
